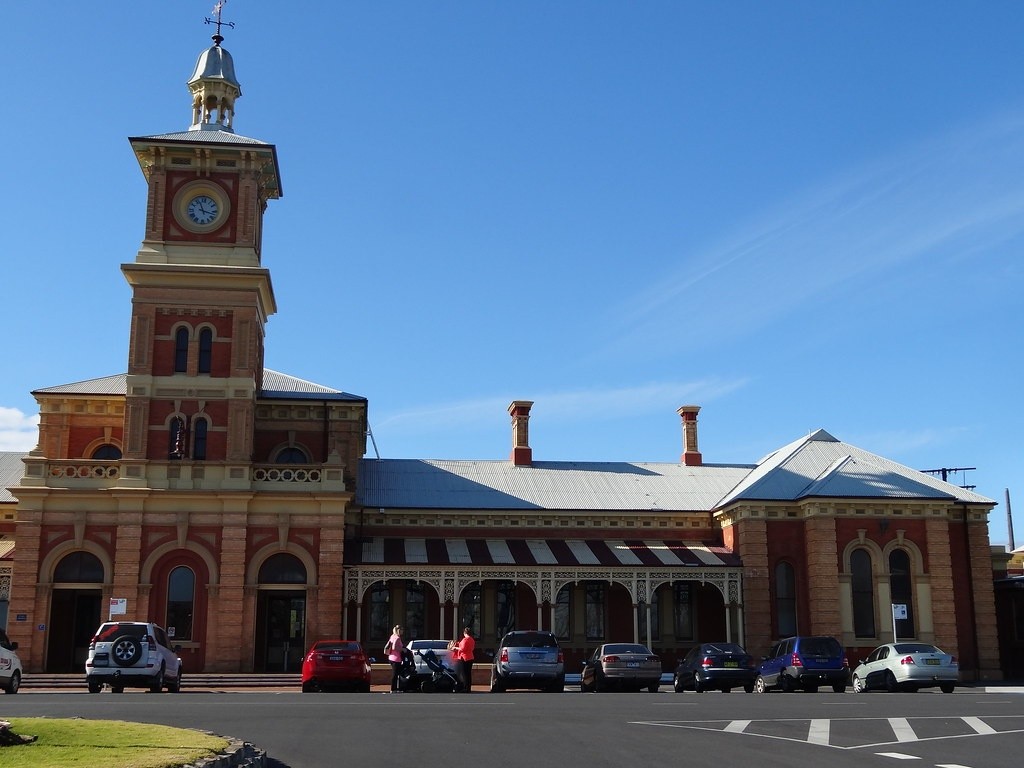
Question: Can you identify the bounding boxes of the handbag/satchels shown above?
[384,641,391,655]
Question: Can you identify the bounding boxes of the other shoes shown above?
[390,690,403,694]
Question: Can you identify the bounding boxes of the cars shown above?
[0,627,22,694]
[300,640,376,693]
[852,643,959,693]
[490,631,565,693]
[581,643,662,693]
[673,642,757,693]
[398,640,462,690]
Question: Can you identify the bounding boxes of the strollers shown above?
[416,648,461,693]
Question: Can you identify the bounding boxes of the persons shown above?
[387,624,407,694]
[448,635,464,680]
[451,627,475,693]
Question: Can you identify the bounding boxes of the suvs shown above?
[757,635,851,692]
[85,621,182,693]
